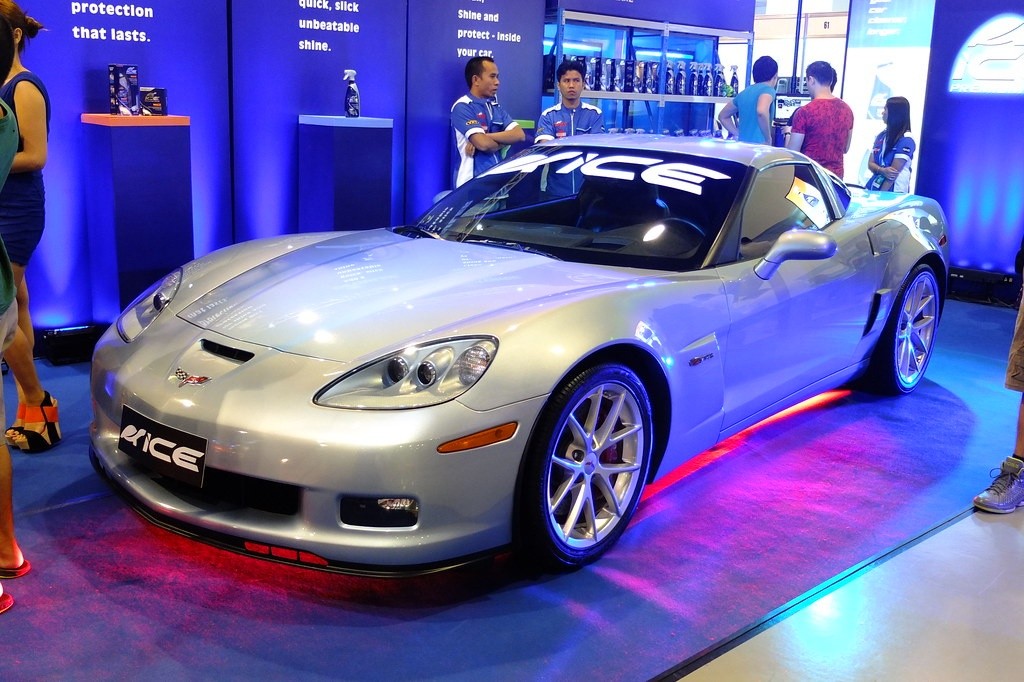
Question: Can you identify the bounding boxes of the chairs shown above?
[568,162,671,233]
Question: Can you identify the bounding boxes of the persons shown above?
[718,56,780,150]
[973,240,1024,514]
[534,62,606,203]
[452,55,525,215]
[783,61,854,182]
[866,96,917,194]
[1,0,58,610]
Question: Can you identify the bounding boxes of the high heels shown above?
[15,390,61,449]
[5,403,28,445]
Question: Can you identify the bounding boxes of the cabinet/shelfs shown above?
[540,7,857,156]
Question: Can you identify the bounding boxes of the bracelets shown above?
[785,132,790,136]
[876,166,880,174]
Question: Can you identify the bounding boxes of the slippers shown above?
[0,594,13,613]
[0,560,31,578]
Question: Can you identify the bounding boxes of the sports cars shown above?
[89,132,950,581]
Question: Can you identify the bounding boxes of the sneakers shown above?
[974,456,1024,514]
